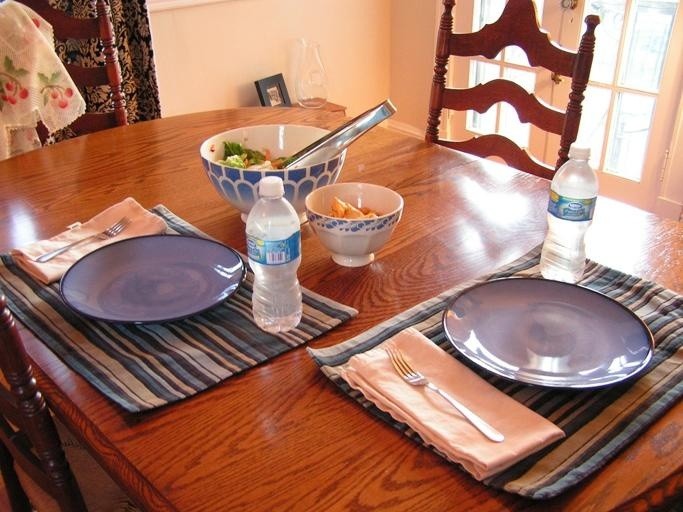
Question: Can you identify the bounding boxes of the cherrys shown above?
[40,86,74,108]
[1,72,29,104]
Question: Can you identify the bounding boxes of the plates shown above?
[441,278,655,389]
[58,235,246,324]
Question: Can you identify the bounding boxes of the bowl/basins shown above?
[304,182,404,268]
[198,123,346,224]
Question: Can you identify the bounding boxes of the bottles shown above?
[539,144,598,283]
[244,175,301,333]
[294,43,330,109]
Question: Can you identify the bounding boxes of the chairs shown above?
[423,1,602,184]
[0,292,90,511]
[16,1,127,156]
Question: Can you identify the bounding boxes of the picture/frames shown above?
[254,73,289,106]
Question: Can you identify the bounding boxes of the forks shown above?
[386,345,504,443]
[36,216,132,265]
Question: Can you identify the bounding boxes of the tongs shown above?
[280,99,396,171]
